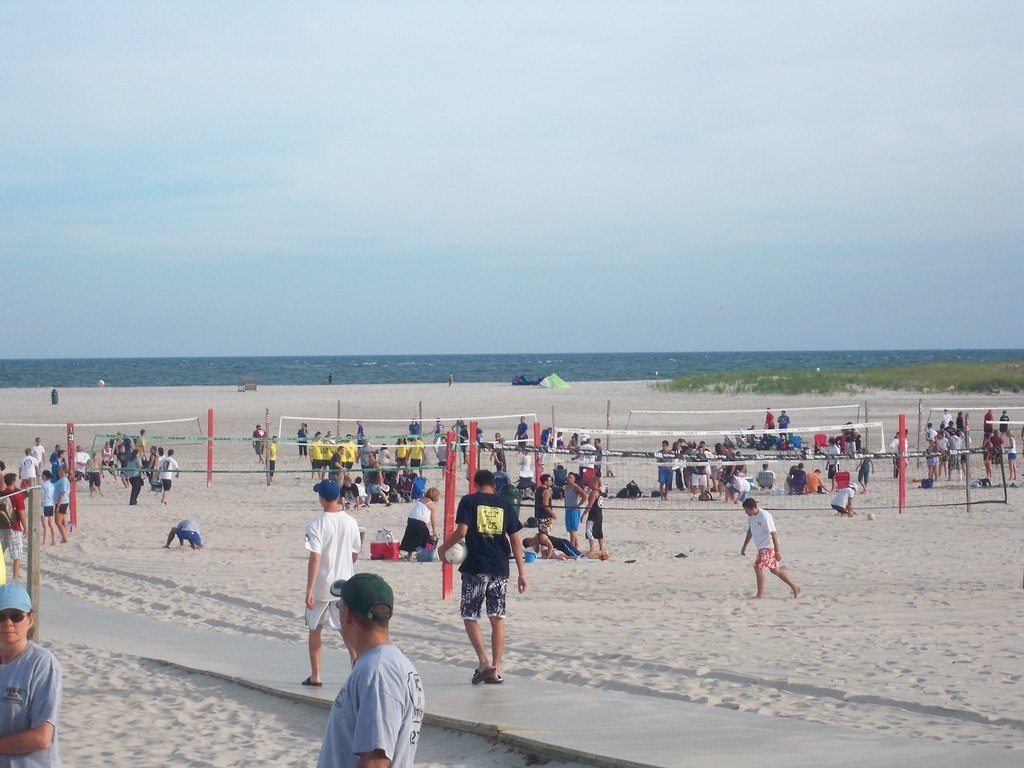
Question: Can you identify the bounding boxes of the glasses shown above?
[0,613,25,623]
[335,600,345,608]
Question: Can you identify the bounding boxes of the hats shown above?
[992,429,1001,434]
[0,582,33,613]
[313,478,340,500]
[330,572,395,621]
[849,483,859,491]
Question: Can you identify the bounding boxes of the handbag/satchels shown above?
[151,481,163,493]
[698,491,712,501]
[921,478,934,488]
[378,529,393,547]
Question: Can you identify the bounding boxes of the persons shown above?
[0,582,63,768]
[656,408,876,505]
[424,416,605,562]
[0,429,179,584]
[399,487,441,561]
[252,425,267,464]
[831,482,858,517]
[328,372,333,385]
[889,409,1024,481]
[266,436,277,482]
[302,479,361,686]
[317,573,425,768]
[438,469,527,684]
[741,498,801,600]
[297,418,427,512]
[448,371,453,386]
[163,519,203,551]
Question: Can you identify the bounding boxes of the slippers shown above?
[302,676,323,686]
[472,665,496,683]
[485,675,504,683]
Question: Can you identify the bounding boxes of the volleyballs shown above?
[867,512,875,520]
[444,539,468,564]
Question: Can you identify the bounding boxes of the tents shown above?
[539,373,570,389]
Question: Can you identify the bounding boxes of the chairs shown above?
[515,466,596,498]
[401,483,414,504]
[758,470,775,493]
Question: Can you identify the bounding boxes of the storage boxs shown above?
[369,527,401,562]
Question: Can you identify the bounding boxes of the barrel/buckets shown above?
[414,547,434,562]
[524,551,536,563]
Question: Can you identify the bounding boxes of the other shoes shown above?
[385,503,391,507]
[690,495,698,500]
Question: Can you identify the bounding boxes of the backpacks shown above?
[617,480,641,499]
[0,492,20,528]
